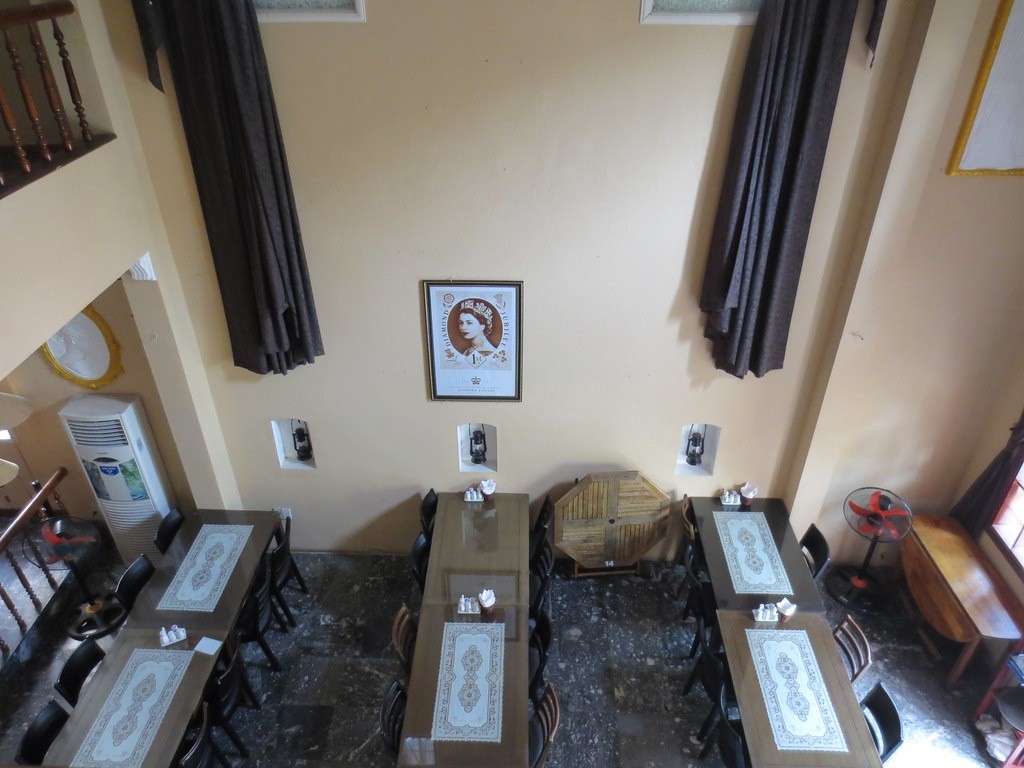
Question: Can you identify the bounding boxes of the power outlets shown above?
[281,506,293,519]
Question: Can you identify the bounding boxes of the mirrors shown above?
[40,303,125,391]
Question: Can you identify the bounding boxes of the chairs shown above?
[531,493,555,563]
[380,680,406,754]
[528,538,555,621]
[529,681,560,768]
[669,494,708,602]
[14,515,309,768]
[832,615,872,684]
[411,532,429,593]
[801,525,832,582]
[858,681,905,764]
[527,608,554,714]
[419,489,439,540]
[679,546,723,655]
[698,685,749,768]
[391,601,419,670]
[683,619,742,741]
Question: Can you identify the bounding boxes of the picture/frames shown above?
[422,279,522,403]
[945,0,1024,177]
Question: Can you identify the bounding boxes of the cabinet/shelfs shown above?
[966,653,1024,768]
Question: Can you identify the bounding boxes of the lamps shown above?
[0,459,20,487]
[0,392,35,482]
[685,423,708,465]
[290,418,313,463]
[468,423,487,464]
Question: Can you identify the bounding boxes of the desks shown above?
[891,513,1024,690]
[681,611,884,768]
[41,628,258,768]
[394,490,530,768]
[121,508,308,706]
[667,497,824,655]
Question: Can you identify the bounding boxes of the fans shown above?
[22,515,128,641]
[825,486,914,610]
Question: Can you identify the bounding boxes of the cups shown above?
[458,595,479,612]
[756,603,778,620]
[722,490,740,503]
[159,625,185,645]
[465,487,483,500]
